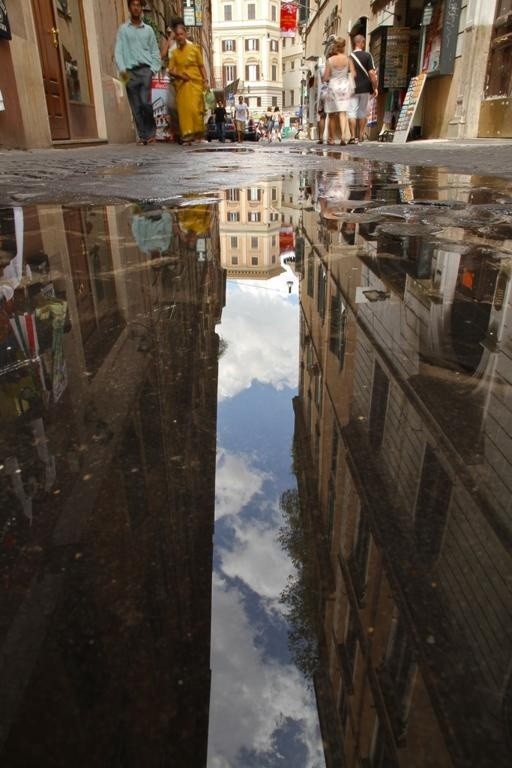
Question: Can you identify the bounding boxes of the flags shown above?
[277,224,294,255]
[279,0,298,38]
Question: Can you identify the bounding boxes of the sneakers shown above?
[146,136,155,144]
[347,139,355,144]
[137,137,145,145]
[359,136,364,141]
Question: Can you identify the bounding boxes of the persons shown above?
[346,33,380,145]
[126,191,219,276]
[207,95,284,144]
[159,16,192,141]
[113,0,162,147]
[307,149,381,238]
[322,37,356,147]
[168,23,209,146]
[315,34,338,144]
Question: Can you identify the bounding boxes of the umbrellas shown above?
[6,267,72,413]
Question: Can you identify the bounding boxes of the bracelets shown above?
[202,78,209,84]
[174,72,177,78]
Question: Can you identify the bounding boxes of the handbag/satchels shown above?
[205,89,217,109]
[347,57,357,96]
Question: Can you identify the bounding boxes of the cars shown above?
[206,114,237,142]
[243,118,263,141]
